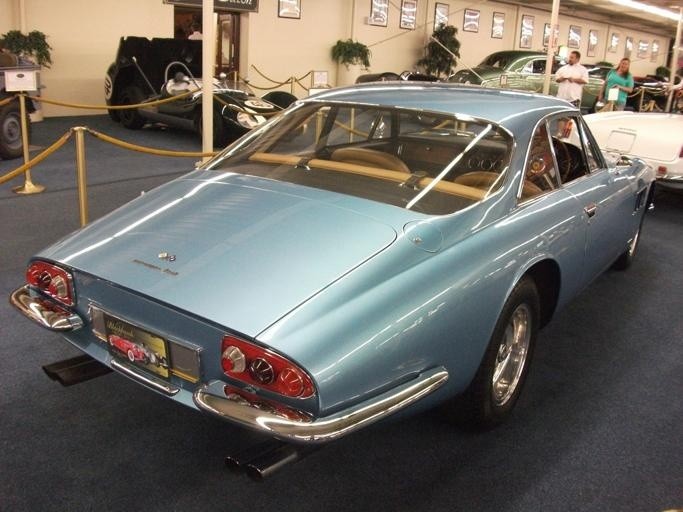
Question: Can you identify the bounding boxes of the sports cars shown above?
[102,36,306,150]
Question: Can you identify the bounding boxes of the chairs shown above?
[328,145,545,203]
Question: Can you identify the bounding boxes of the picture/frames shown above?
[369,1,599,59]
[275,1,302,21]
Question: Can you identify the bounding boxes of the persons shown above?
[555,51,589,109]
[598,58,634,111]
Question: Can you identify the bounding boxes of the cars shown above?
[9,77,655,483]
[0,47,36,161]
[347,36,683,202]
